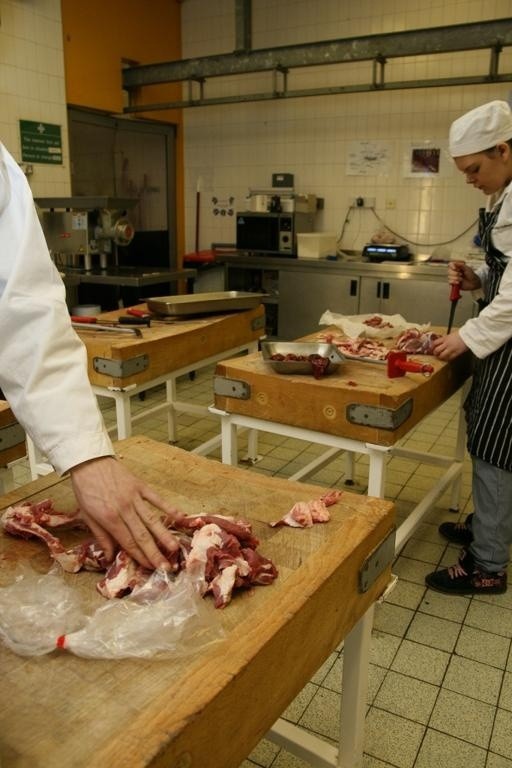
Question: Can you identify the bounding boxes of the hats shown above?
[448,99,512,159]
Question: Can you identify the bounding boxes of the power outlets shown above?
[346,197,375,208]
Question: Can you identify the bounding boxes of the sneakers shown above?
[438,513,474,545]
[424,548,507,596]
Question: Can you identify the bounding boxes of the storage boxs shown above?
[296,232,337,259]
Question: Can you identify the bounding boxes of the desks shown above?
[69,306,265,466]
[2,399,29,489]
[209,313,475,568]
[1,435,397,766]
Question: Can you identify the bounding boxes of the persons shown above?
[0,140,185,574]
[425,99,511,596]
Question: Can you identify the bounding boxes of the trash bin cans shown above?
[184,259,241,294]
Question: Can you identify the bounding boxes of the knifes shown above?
[447,262,464,336]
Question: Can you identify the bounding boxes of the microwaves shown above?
[234,209,312,260]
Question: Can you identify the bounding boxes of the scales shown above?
[361,243,409,260]
